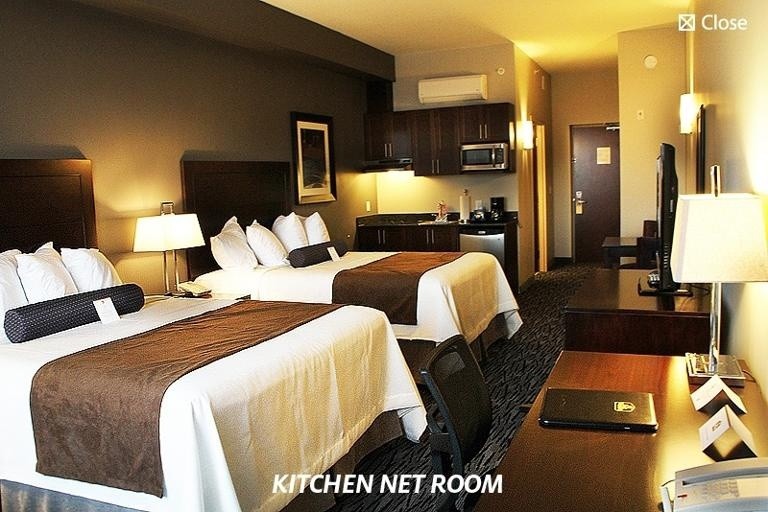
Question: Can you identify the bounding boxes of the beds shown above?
[0,160,427,512]
[180,161,522,387]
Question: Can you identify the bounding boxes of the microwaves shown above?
[460,143,510,171]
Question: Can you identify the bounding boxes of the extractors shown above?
[360,158,412,172]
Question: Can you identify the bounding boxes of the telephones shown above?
[177,280,212,297]
[673,457,768,512]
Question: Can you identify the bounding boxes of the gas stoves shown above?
[366,215,419,225]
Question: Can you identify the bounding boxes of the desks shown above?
[472,351,767,511]
[565,266,726,353]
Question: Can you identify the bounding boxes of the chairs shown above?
[601,221,662,267]
[420,332,500,512]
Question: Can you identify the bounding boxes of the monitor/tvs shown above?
[637,142,694,298]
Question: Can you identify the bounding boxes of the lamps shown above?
[671,191,768,386]
[520,122,535,150]
[133,213,208,298]
[679,93,701,134]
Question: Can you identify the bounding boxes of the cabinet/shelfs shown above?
[356,213,516,300]
[367,103,516,176]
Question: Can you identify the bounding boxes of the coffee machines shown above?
[490,196,504,223]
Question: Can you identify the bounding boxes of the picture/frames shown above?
[291,111,338,206]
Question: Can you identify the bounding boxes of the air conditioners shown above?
[418,74,487,104]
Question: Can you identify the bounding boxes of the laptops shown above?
[538,387,658,434]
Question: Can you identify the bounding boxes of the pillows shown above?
[0,242,144,342]
[208,213,348,270]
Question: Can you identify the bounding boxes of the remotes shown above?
[647,273,661,287]
